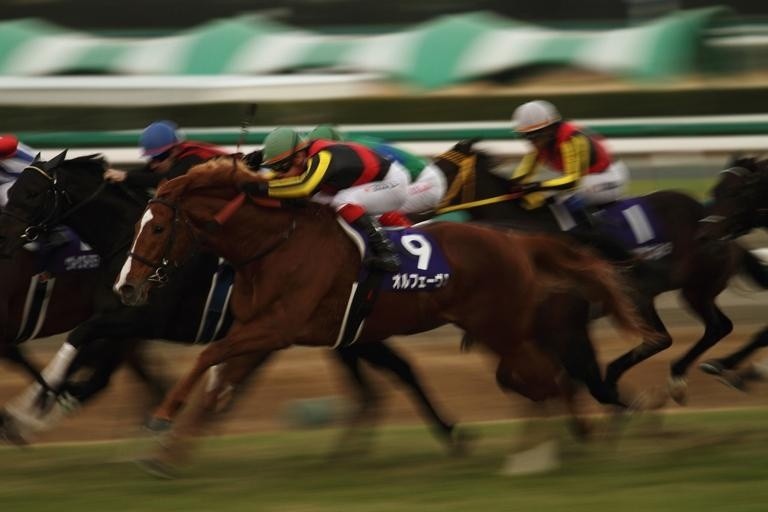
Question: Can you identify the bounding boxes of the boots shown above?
[569,206,610,246]
[349,211,401,279]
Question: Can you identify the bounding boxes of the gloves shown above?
[511,181,541,193]
[244,180,268,197]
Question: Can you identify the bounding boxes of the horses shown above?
[1,134,767,485]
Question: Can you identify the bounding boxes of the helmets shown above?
[510,99,565,135]
[304,127,342,143]
[138,123,185,161]
[259,127,310,167]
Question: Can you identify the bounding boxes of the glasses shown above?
[153,150,171,162]
[271,155,294,172]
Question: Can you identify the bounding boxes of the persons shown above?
[505,99,632,243]
[102,113,235,205]
[0,135,44,207]
[260,120,450,275]
